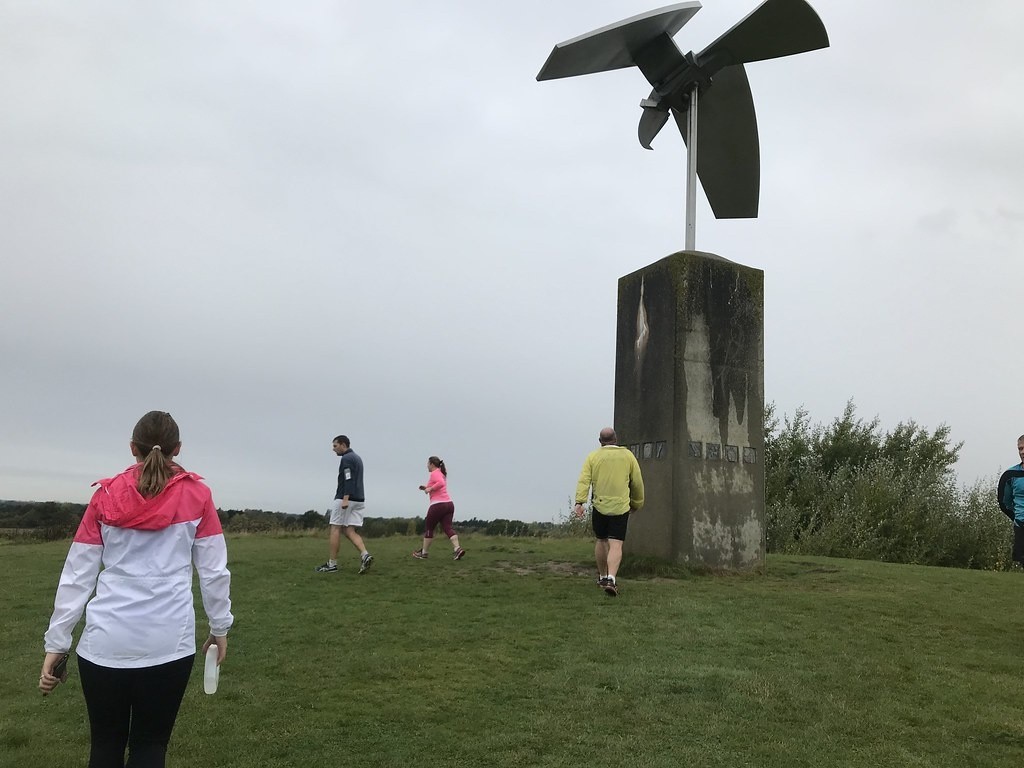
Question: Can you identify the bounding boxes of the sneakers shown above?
[596,577,608,587]
[454,548,465,560]
[604,578,620,596]
[413,549,428,559]
[358,554,373,574]
[315,564,338,573]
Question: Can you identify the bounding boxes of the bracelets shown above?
[575,503,583,505]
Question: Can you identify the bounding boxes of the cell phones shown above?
[43,653,70,697]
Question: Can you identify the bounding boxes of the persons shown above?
[40,410,234,768]
[413,456,465,560]
[574,428,645,594]
[998,435,1024,566]
[315,435,373,573]
